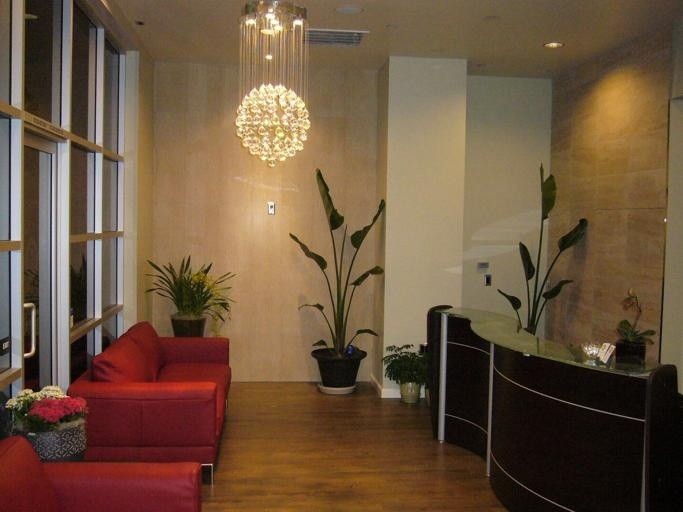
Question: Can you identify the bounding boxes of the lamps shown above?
[235,0,310,166]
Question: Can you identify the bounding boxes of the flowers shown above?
[144,254,238,337]
[4,386,90,430]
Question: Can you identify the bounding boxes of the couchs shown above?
[67,321,232,491]
[2,437,205,511]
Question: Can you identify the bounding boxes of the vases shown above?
[23,425,88,461]
[171,311,207,337]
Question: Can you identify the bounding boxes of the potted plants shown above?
[613,290,656,363]
[380,341,424,404]
[290,168,382,392]
